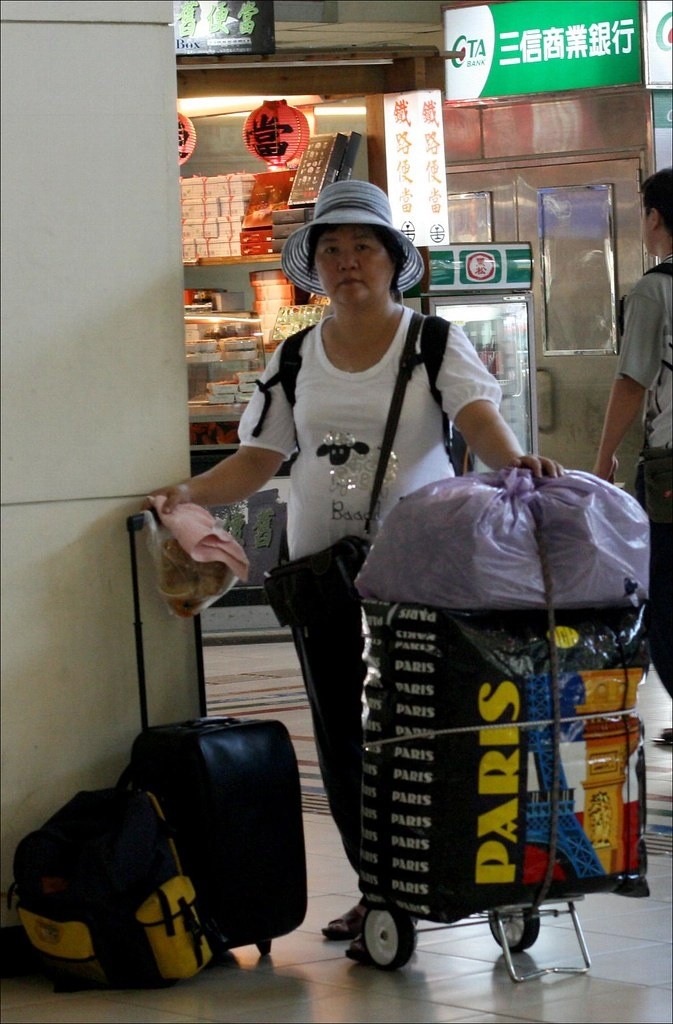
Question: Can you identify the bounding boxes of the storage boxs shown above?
[179,125,363,405]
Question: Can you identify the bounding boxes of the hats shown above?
[280,180,426,293]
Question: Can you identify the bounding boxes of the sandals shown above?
[344,932,372,964]
[321,897,372,940]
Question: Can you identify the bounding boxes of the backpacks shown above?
[7,761,218,992]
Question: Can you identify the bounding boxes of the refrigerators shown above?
[400,239,539,477]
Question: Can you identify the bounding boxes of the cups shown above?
[157,577,200,618]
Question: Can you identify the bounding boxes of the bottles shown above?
[463,329,497,376]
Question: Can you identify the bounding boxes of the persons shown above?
[589,168,673,745]
[141,181,565,963]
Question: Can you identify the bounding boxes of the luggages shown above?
[127,512,307,956]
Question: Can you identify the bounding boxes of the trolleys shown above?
[360,895,590,984]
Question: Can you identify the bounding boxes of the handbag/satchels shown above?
[355,461,652,925]
[264,534,372,628]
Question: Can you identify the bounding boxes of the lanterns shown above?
[243,99,310,172]
[178,113,197,167]
